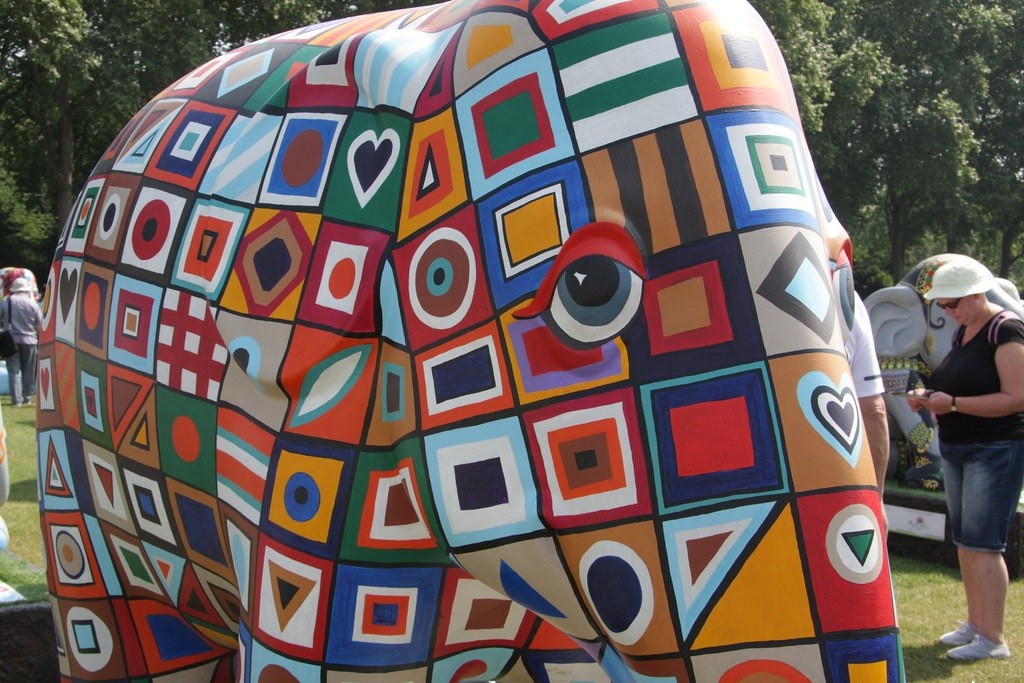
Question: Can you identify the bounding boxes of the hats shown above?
[9,278,30,292]
[923,257,994,299]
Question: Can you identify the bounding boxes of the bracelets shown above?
[950,397,958,413]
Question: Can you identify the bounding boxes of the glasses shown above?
[936,297,961,309]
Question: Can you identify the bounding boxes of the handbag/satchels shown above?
[0,331,16,359]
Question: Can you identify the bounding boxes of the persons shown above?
[844,290,892,536]
[907,258,1023,661]
[0,278,42,406]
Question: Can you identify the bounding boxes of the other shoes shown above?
[22,397,31,405]
[14,401,22,407]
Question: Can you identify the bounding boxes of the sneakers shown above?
[946,634,1011,661]
[939,620,981,646]
